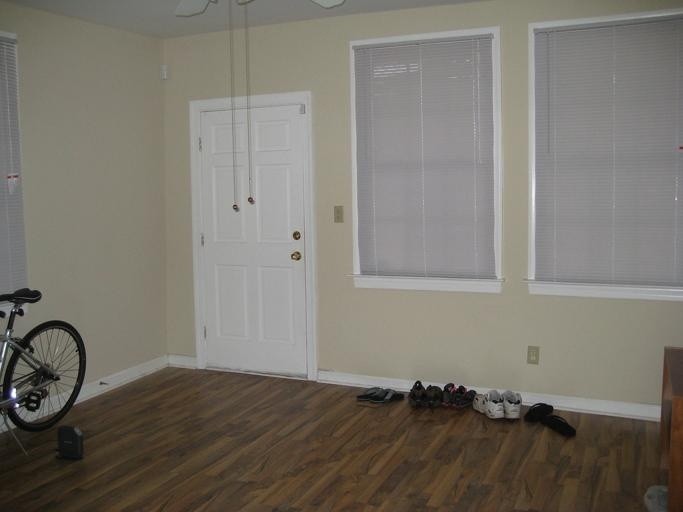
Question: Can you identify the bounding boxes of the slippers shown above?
[540,415,575,437]
[356,386,384,401]
[523,403,552,423]
[369,389,405,405]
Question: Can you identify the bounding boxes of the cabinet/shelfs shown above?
[658,342,682,512]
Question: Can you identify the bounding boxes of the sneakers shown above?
[407,381,521,420]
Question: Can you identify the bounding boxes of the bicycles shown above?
[0,286,86,456]
